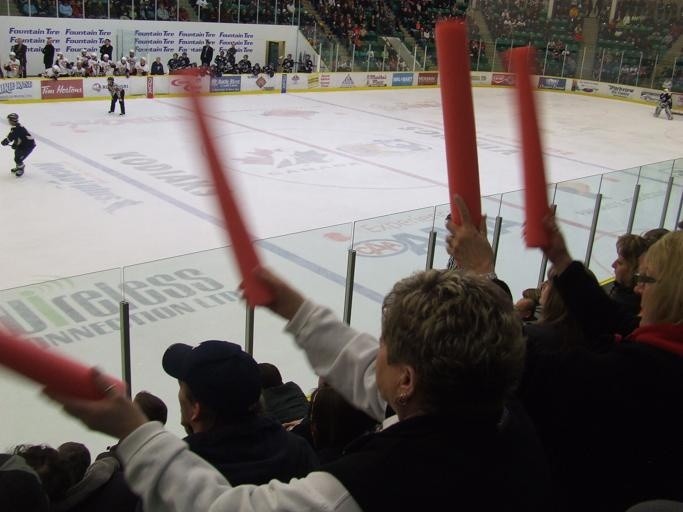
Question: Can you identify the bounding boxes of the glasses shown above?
[632,269,658,288]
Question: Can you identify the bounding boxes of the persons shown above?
[106,77,128,114]
[653,87,674,121]
[1,1,682,92]
[1,113,34,176]
[0,220,682,510]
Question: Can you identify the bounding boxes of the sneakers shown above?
[108,111,125,115]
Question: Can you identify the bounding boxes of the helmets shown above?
[56,51,63,60]
[90,53,96,59]
[173,50,311,71]
[140,57,146,63]
[9,51,15,56]
[103,54,108,60]
[129,48,134,55]
[76,60,83,66]
[53,65,60,74]
[107,77,114,81]
[121,57,126,63]
[81,50,86,55]
[7,113,18,121]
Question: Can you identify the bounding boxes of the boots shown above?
[11,165,25,176]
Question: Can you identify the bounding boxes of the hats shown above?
[259,362,282,384]
[162,339,263,416]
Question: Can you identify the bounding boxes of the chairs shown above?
[347,0,683,92]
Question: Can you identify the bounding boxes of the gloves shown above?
[1,138,9,146]
